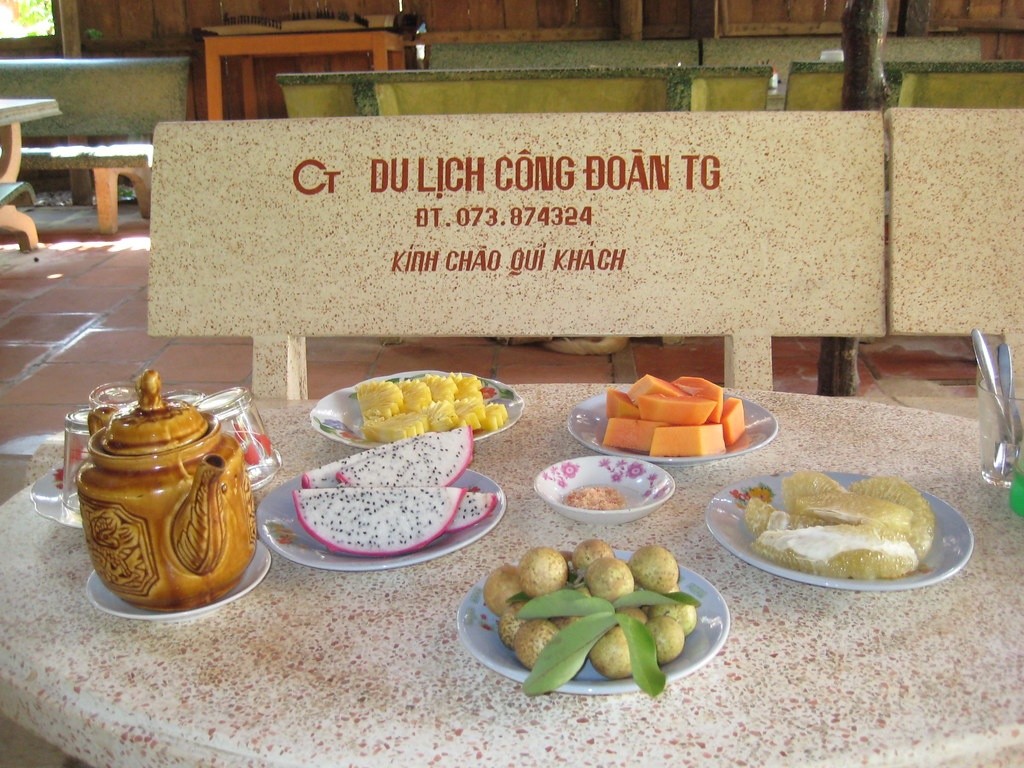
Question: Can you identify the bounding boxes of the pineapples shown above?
[355,371,507,444]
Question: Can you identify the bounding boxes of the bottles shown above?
[1009,436,1023,518]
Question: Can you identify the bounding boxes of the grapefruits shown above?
[743,468,934,580]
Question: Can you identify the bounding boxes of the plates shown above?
[256,468,507,571]
[30,458,84,529]
[457,550,731,696]
[705,474,975,591]
[567,386,779,465]
[310,370,525,449]
[85,540,271,622]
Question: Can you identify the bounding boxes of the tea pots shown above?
[78,369,256,613]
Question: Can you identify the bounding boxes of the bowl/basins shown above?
[533,456,676,525]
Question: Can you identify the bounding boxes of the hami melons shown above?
[600,373,748,458]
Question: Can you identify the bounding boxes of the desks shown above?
[203,27,407,120]
[0,99,64,252]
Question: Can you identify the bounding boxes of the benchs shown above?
[0,107,1024,767]
[0,57,191,234]
[276,36,1024,119]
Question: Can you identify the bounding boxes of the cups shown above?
[977,374,1024,488]
[88,380,139,412]
[61,408,92,512]
[193,385,278,483]
[162,388,206,406]
